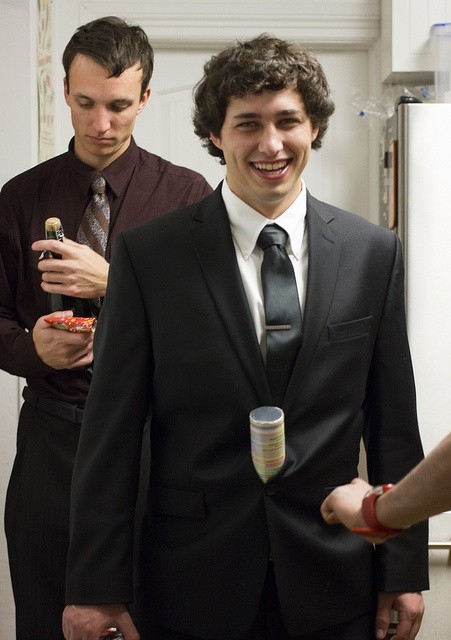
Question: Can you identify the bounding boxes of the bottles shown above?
[44,218,92,385]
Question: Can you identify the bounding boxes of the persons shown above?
[319,431,450,544]
[61,31,431,639]
[1,15,219,640]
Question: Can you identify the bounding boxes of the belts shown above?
[22,385,84,425]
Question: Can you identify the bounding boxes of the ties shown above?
[256,224,302,409]
[76,176,111,321]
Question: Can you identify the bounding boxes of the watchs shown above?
[351,483,405,537]
